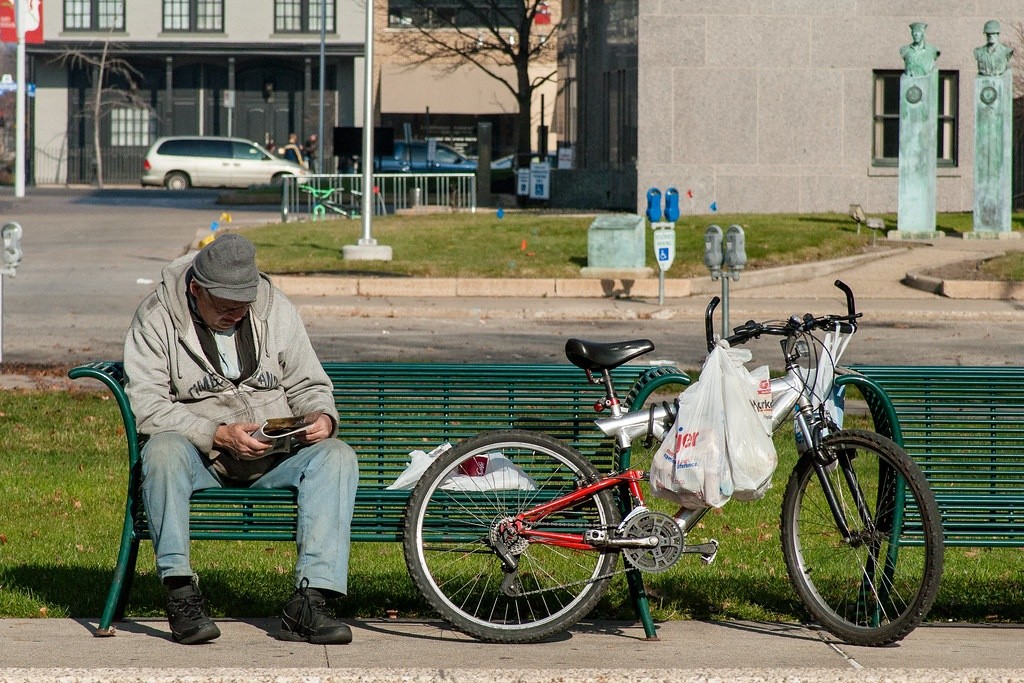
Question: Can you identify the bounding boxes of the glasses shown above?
[204,284,252,315]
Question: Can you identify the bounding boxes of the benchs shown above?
[833,364,1024,628]
[68,360,692,642]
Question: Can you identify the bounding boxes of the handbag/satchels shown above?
[649,344,775,509]
[791,322,855,472]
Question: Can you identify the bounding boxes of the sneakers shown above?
[279,576,352,646]
[165,587,221,645]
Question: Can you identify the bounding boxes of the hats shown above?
[192,235,258,302]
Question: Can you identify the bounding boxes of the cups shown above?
[458,457,488,477]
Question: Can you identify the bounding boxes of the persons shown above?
[262,138,277,160]
[283,133,319,174]
[121,234,362,644]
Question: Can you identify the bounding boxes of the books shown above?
[237,408,325,461]
